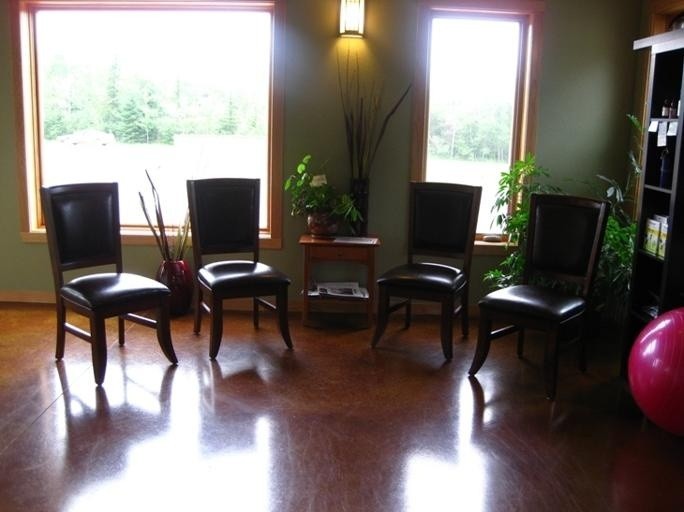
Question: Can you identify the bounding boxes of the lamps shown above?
[336,1,366,40]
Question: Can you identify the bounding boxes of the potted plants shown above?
[135,170,193,315]
[333,37,411,234]
[281,155,361,241]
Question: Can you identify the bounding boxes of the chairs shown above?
[39,181,177,385]
[186,178,294,358]
[370,182,483,359]
[468,193,610,402]
[51,349,178,486]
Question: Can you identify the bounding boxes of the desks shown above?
[298,234,381,327]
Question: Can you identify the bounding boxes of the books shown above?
[640,212,668,259]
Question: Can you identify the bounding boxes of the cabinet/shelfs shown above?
[615,27,683,423]
